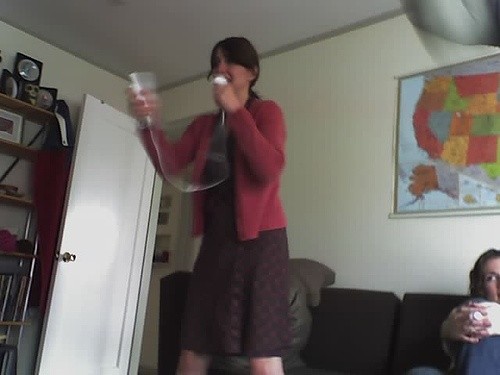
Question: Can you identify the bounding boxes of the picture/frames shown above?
[388,52,500,216]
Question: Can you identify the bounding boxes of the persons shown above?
[439,248,500,375]
[122,35,290,375]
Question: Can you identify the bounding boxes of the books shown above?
[1,344,17,375]
[0,273,30,322]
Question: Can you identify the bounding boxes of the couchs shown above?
[159,271,468,374]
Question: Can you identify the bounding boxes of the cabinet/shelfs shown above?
[1,92,54,374]
